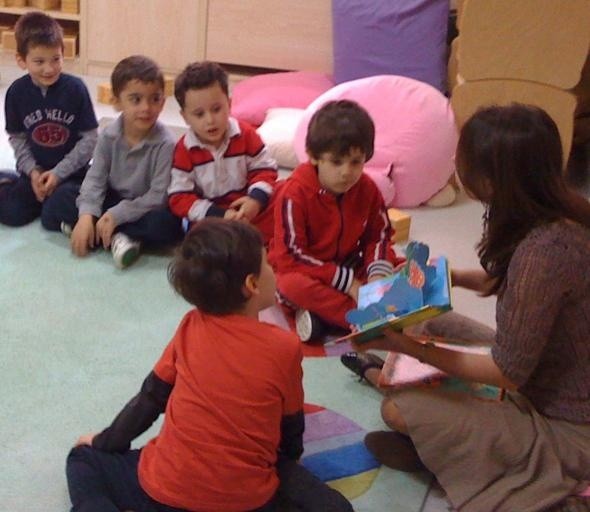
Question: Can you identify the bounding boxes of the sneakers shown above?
[295,308,326,343]
[109,232,141,269]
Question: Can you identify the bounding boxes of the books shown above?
[324,240,452,348]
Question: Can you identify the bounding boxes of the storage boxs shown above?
[0,1,25,7]
[0,24,14,42]
[60,0,80,15]
[98,73,175,104]
[28,1,60,11]
[2,28,18,51]
[61,30,78,58]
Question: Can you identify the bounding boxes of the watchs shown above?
[416,341,435,363]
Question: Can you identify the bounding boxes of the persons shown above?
[0,12,99,232]
[348,102,589,512]
[64,221,305,512]
[266,99,412,344]
[167,61,287,250]
[57,56,179,268]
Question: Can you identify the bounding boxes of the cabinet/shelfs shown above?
[448,0,590,191]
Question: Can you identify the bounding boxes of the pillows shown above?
[294,75,460,209]
[332,0,451,95]
[231,71,335,127]
[255,108,304,169]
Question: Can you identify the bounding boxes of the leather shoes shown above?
[340,350,394,389]
[364,430,430,473]
